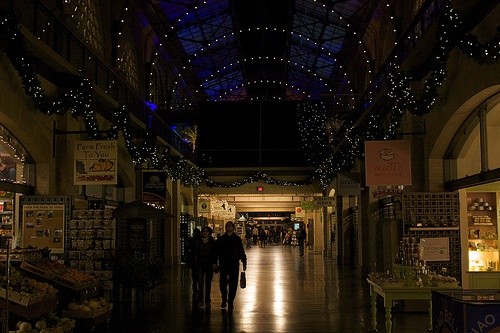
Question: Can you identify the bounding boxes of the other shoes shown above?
[221,302,233,308]
[206,302,209,307]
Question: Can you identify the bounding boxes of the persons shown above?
[216,222,247,310]
[191,226,218,308]
[246,224,292,248]
[296,224,306,256]
[188,229,202,295]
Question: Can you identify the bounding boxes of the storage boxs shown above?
[0,260,114,329]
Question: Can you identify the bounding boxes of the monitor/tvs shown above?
[293,222,301,231]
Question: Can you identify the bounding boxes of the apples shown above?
[68,297,106,311]
[60,269,95,282]
[0,265,56,298]
[16,320,48,333]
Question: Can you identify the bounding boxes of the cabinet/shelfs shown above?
[432,290,500,333]
[459,189,500,289]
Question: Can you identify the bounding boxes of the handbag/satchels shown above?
[240,272,246,288]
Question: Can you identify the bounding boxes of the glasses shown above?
[202,229,208,232]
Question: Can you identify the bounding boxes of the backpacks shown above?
[296,230,302,238]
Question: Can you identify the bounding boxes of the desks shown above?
[365,275,458,332]
